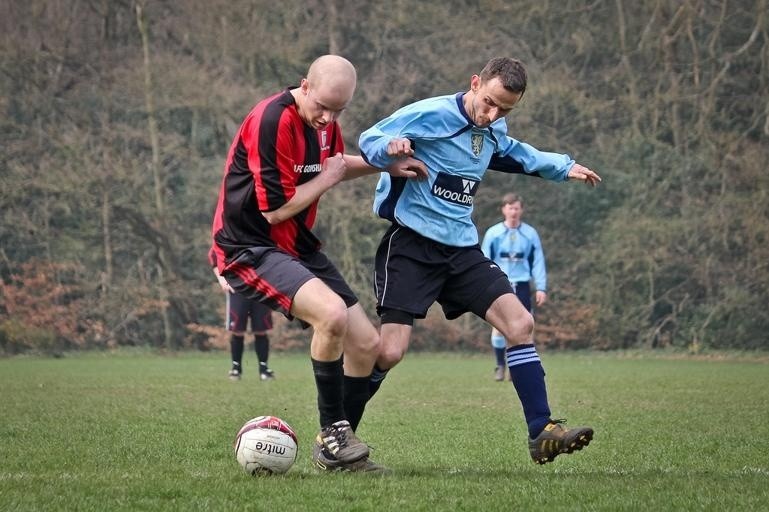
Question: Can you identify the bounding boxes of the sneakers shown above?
[495,366,513,381]
[259,362,275,380]
[312,420,392,477]
[528,422,593,465]
[228,360,241,381]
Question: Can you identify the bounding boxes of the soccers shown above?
[235,416,298,475]
